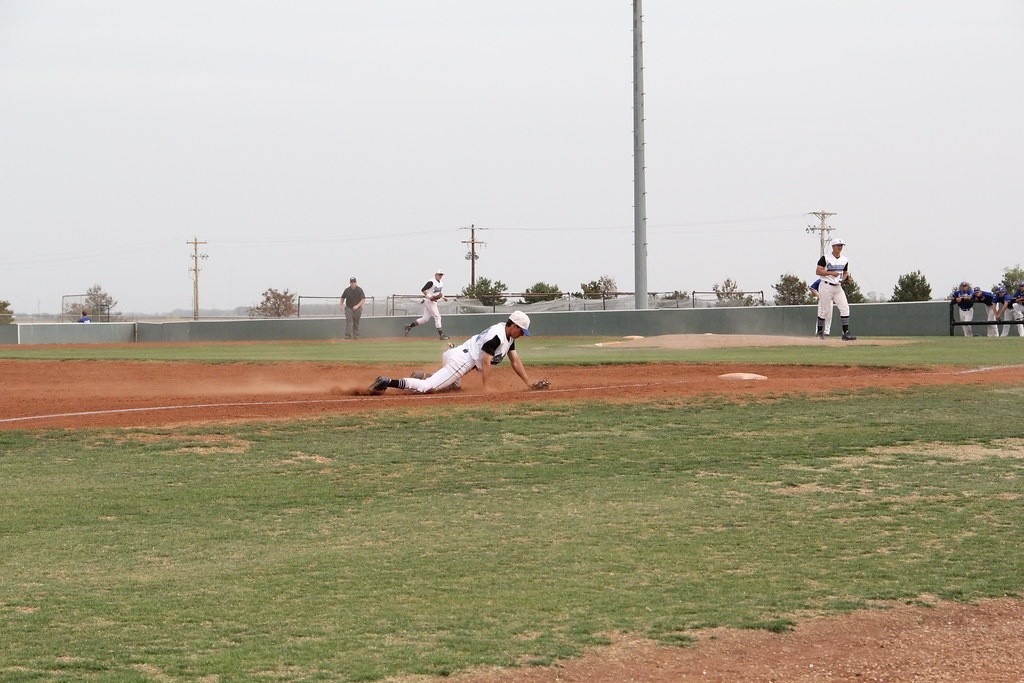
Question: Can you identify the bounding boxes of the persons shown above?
[369,311,551,394]
[810,239,857,341]
[404,270,451,340]
[954,282,974,336]
[972,287,999,336]
[1013,282,1024,321]
[992,286,1024,336]
[340,277,365,339]
[78,310,91,323]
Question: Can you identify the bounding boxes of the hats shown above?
[998,287,1005,292]
[509,310,531,336]
[832,238,845,246]
[974,287,980,292]
[435,270,444,275]
[350,277,356,283]
[1020,282,1024,286]
[961,282,968,286]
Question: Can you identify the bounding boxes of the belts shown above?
[463,349,469,353]
[825,281,839,286]
[961,308,970,311]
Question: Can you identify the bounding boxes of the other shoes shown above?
[405,323,411,337]
[411,371,424,380]
[439,334,451,340]
[367,376,391,391]
[842,330,857,341]
[817,331,825,340]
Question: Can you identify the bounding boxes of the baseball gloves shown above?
[434,293,442,299]
[839,275,854,285]
[532,378,550,390]
